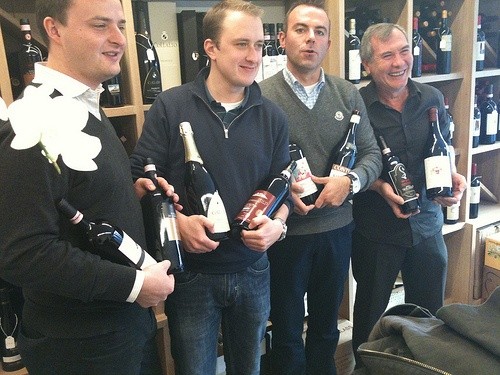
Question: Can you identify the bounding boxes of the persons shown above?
[351,24,468,374]
[256,1,383,375]
[131,1,295,374]
[0,0,176,375]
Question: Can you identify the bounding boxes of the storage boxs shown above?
[482,265,500,300]
[484,232,500,270]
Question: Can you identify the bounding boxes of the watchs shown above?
[346,174,361,195]
[274,217,288,241]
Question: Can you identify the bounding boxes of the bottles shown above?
[444,201,461,225]
[473,76,500,148]
[100,71,124,107]
[53,196,158,273]
[445,96,453,144]
[424,107,453,201]
[288,141,320,206]
[469,163,481,219]
[136,8,162,104]
[414,0,453,43]
[17,19,43,91]
[326,109,363,178]
[345,19,362,84]
[481,15,498,38]
[437,11,452,74]
[141,157,186,277]
[231,159,297,242]
[376,135,420,214]
[412,17,422,77]
[476,15,486,71]
[178,121,234,242]
[263,22,285,56]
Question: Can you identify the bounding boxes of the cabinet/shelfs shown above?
[0,0,500,375]
